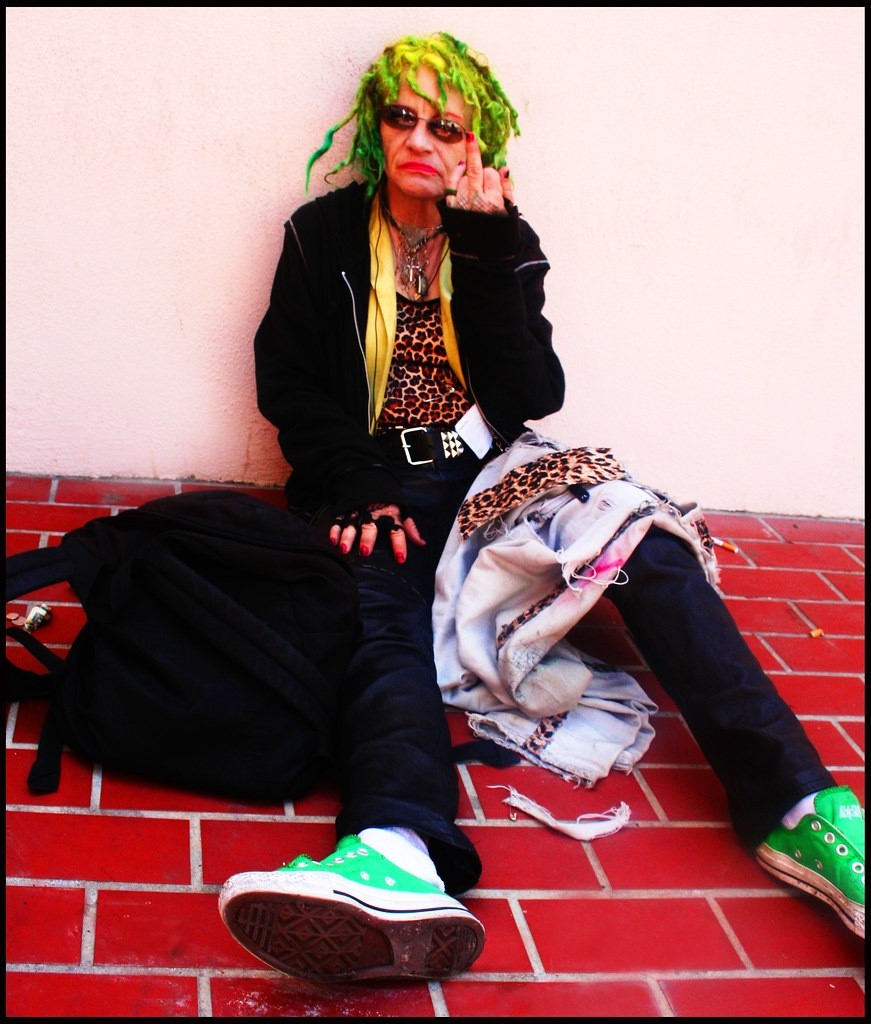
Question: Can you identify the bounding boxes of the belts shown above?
[373,427,509,470]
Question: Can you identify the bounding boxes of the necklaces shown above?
[384,202,451,304]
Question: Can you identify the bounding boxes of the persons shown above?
[218,33,865,984]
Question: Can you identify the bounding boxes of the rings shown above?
[445,187,458,196]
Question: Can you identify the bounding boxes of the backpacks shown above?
[1,487,360,806]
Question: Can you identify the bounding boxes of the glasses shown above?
[376,105,470,144]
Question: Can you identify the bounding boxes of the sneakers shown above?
[217,834,486,981]
[754,785,865,938]
